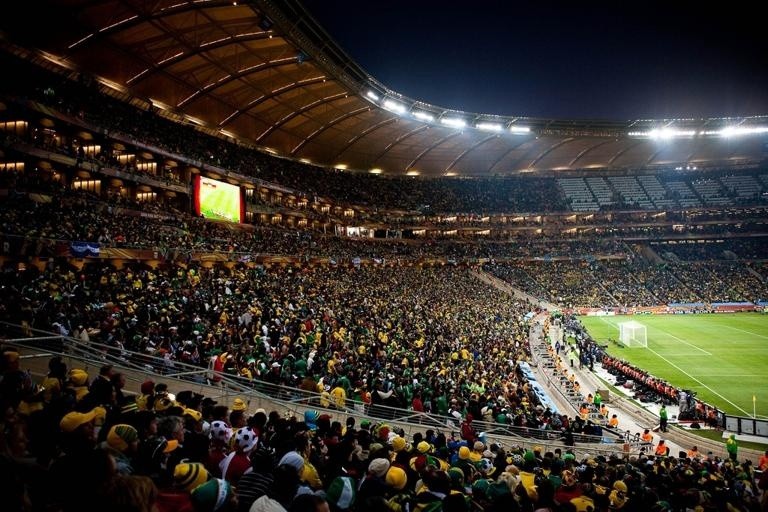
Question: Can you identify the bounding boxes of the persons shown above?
[1,46,768,511]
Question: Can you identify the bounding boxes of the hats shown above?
[173,399,305,511]
[60,412,94,430]
[106,425,137,451]
[361,419,431,490]
[5,351,19,362]
[442,441,508,500]
[328,477,355,509]
[550,448,627,493]
[71,369,88,385]
[510,445,542,464]
[305,411,332,421]
